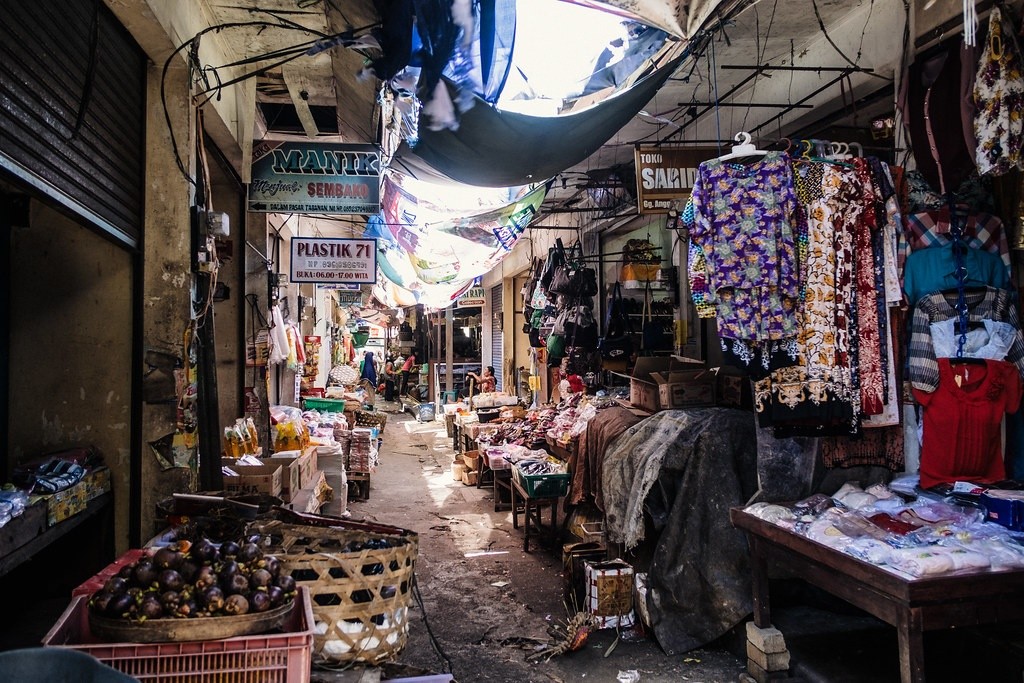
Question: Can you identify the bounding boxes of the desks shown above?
[477,454,558,552]
[453,422,521,455]
[730,502,1024,683]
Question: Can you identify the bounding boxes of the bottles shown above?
[224,418,258,457]
[275,423,310,454]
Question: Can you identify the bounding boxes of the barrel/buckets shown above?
[418,403,435,421]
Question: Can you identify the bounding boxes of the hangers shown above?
[941,193,985,365]
[718,131,870,166]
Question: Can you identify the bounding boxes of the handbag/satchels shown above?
[520,239,604,380]
[641,277,664,352]
[604,281,637,341]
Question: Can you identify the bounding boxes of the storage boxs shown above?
[0,467,112,558]
[441,396,520,416]
[477,437,574,498]
[39,550,315,683]
[223,446,319,503]
[609,354,745,412]
[399,341,416,354]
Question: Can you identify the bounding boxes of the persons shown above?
[468,366,497,393]
[375,350,385,395]
[384,352,405,402]
[360,351,377,411]
[400,348,419,398]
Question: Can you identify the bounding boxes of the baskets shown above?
[238,511,418,666]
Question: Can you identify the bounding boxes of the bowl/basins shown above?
[327,387,345,398]
